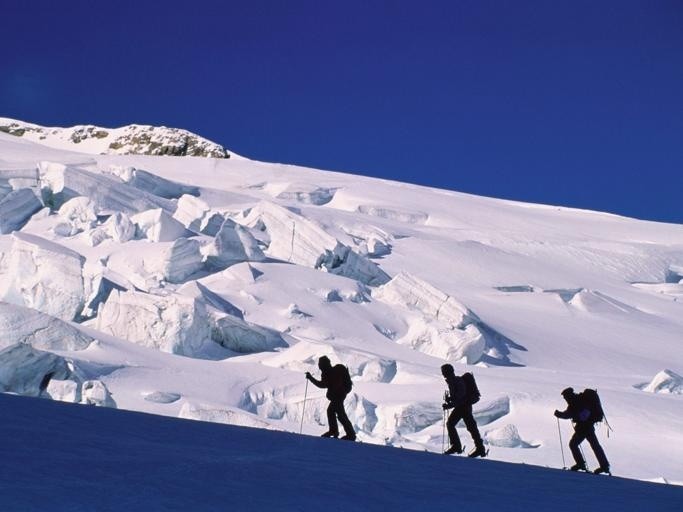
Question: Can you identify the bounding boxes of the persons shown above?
[439,363,485,458]
[554,386,612,475]
[303,355,357,441]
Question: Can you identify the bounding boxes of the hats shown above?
[560,387,574,396]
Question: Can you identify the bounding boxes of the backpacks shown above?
[331,364,352,394]
[579,388,605,423]
[462,374,480,406]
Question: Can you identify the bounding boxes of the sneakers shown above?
[321,430,356,441]
[444,448,487,458]
[570,464,610,477]
